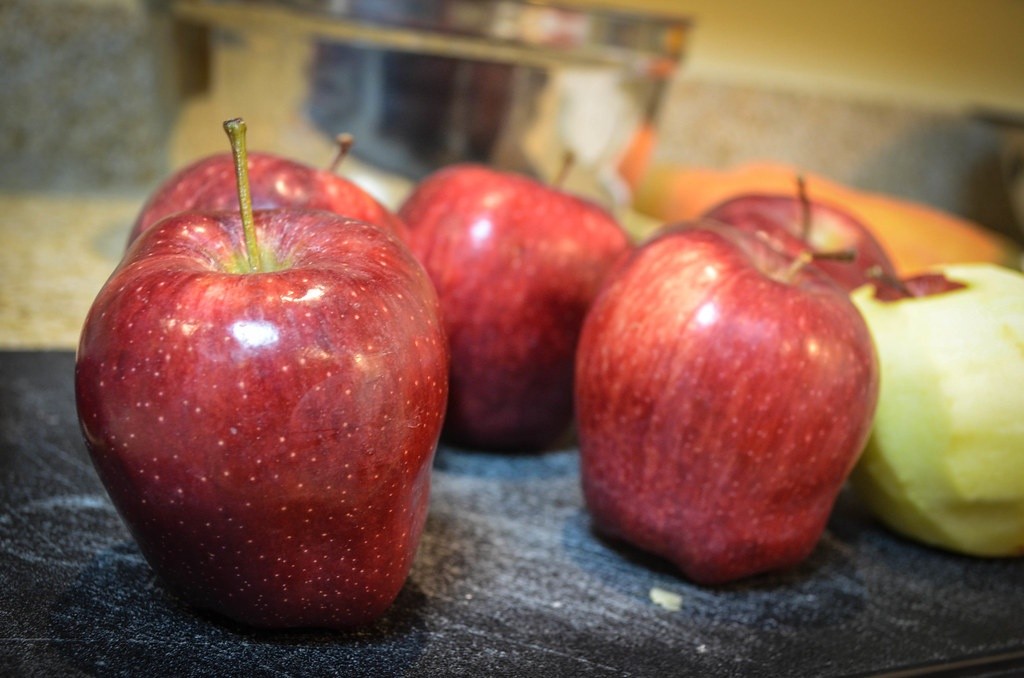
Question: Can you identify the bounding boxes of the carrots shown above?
[624,162,1010,276]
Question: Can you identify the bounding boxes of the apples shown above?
[73,118,1024,639]
